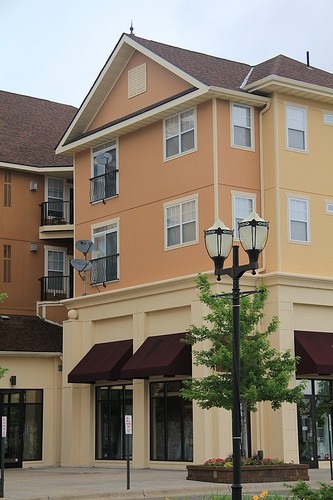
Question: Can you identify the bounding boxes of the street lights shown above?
[203,208,271,500]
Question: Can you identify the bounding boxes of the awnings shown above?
[119,331,192,379]
[67,339,133,383]
[294,330,333,375]
